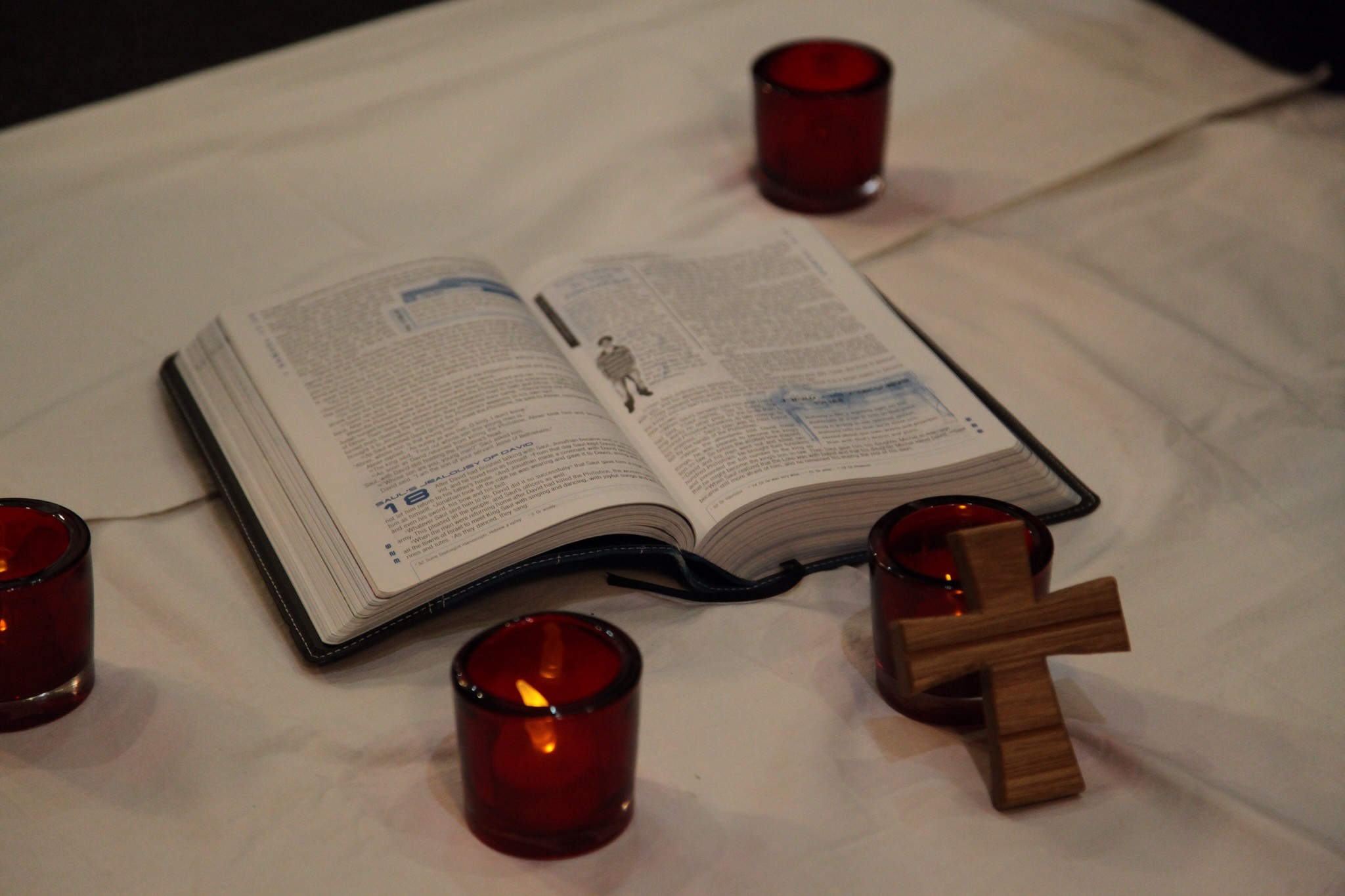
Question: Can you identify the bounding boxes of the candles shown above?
[784,110,859,191]
[491,676,596,795]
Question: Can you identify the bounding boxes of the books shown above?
[160,213,1101,668]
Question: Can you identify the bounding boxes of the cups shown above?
[752,37,894,217]
[1,497,98,733]
[866,492,1054,727]
[449,610,640,861]
